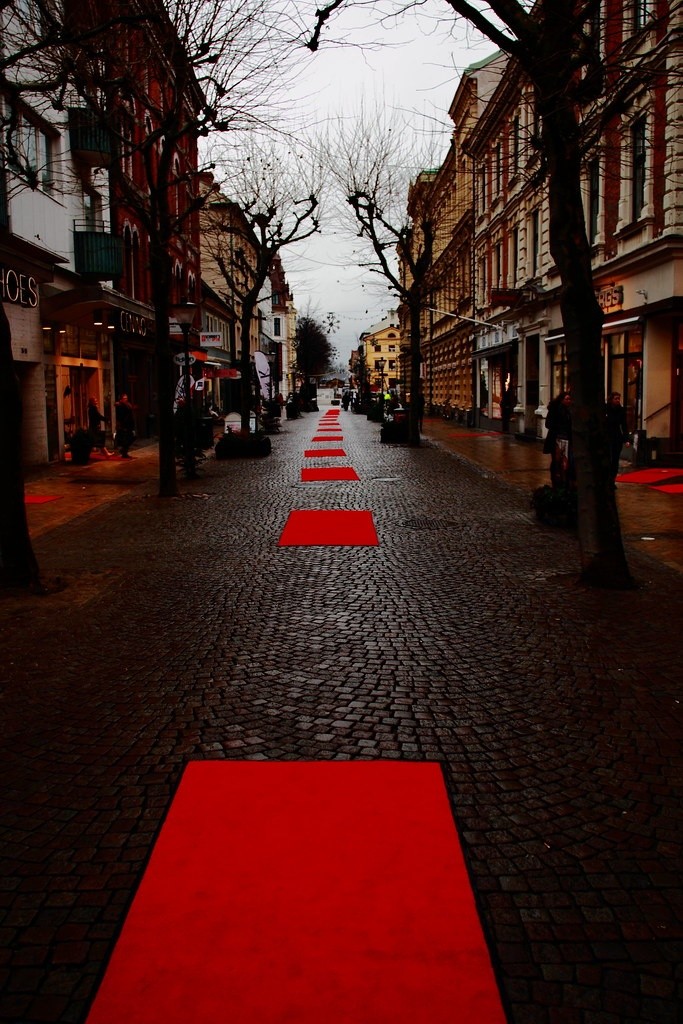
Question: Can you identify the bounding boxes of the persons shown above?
[389,387,406,410]
[87,397,115,456]
[112,393,138,457]
[545,391,632,492]
[341,390,351,411]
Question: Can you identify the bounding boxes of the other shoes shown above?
[122,453,132,458]
[119,449,125,455]
[106,452,115,459]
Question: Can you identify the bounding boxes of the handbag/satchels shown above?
[88,431,105,449]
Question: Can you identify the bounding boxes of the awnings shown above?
[208,363,238,379]
[177,350,221,370]
